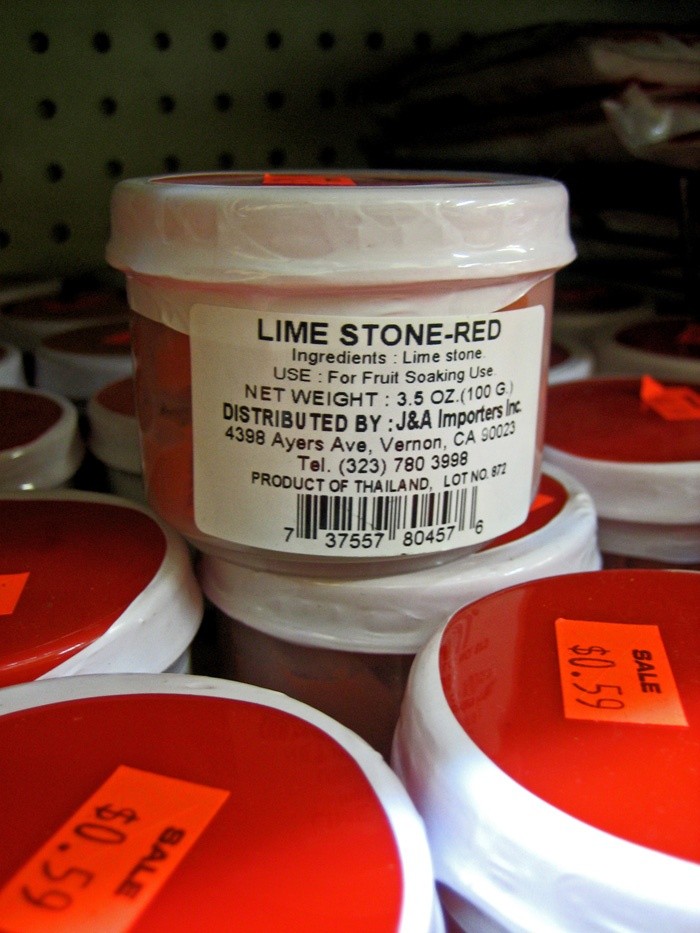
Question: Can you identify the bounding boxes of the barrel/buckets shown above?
[194,462,603,767]
[392,570,700,933]
[547,287,700,389]
[106,169,578,579]
[0,297,145,503]
[0,672,449,933]
[0,384,84,493]
[542,376,700,571]
[0,493,203,690]
